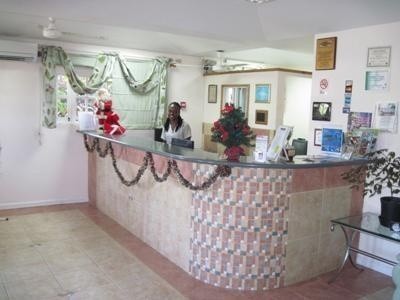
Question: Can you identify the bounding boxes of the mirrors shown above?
[220,84,250,124]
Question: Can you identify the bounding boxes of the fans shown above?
[198,50,249,72]
[34,17,104,40]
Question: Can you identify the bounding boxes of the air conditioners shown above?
[0,39,38,62]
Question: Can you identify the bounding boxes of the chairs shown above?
[291,138,307,155]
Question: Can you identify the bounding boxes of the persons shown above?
[159,101,192,145]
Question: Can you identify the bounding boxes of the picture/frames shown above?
[255,83,271,103]
[315,37,336,70]
[314,129,323,146]
[312,102,331,121]
[208,85,217,103]
[255,110,268,124]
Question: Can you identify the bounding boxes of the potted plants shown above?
[340,137,400,228]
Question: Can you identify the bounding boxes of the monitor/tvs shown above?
[172,138,194,149]
